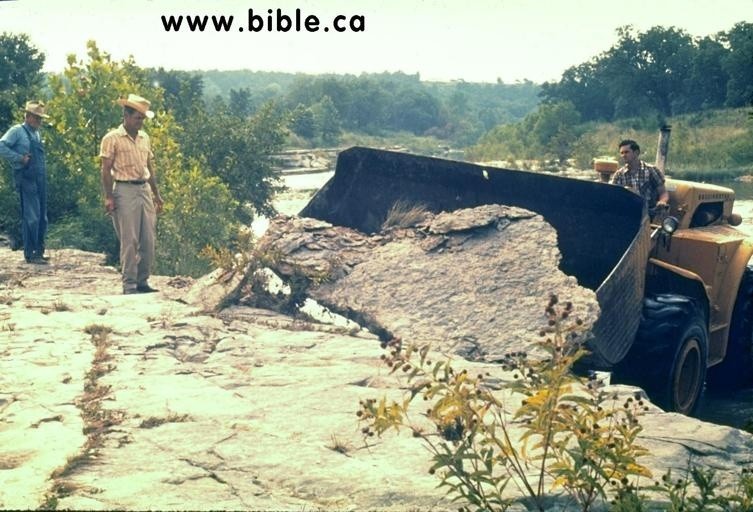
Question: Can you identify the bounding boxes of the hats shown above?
[117,94,154,119]
[19,99,50,119]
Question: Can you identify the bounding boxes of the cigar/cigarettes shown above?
[104,206,118,217]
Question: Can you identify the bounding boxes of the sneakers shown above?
[136,285,160,293]
[123,289,144,294]
[24,254,50,264]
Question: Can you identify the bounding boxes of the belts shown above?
[114,179,146,185]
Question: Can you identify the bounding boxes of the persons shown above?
[0,100,50,264]
[99,93,164,294]
[608,140,670,223]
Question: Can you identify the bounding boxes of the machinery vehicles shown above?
[244,126,751,423]
